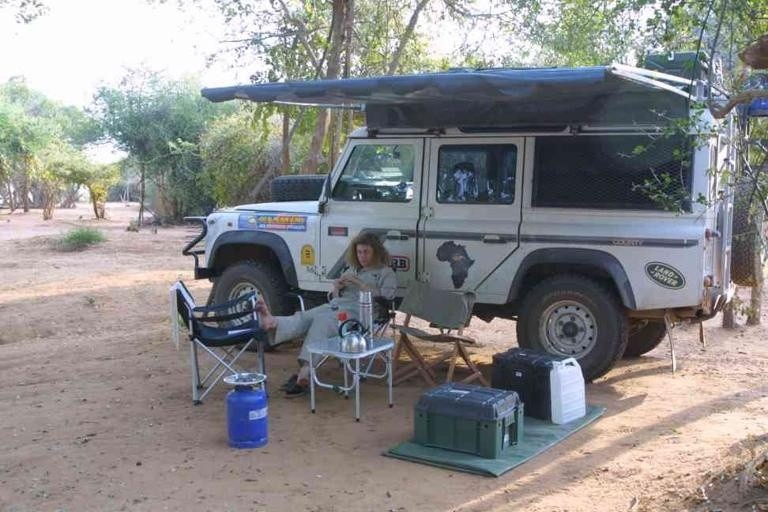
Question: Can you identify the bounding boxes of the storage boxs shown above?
[414,344,587,460]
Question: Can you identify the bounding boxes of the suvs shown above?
[180,65,737,386]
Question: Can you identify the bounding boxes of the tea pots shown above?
[338,318,371,353]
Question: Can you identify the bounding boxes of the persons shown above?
[253,231,396,396]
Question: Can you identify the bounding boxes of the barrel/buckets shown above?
[226,384,268,449]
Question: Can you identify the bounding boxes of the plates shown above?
[224,373,267,386]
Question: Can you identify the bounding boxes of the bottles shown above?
[337,291,375,340]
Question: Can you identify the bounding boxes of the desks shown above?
[305,334,395,422]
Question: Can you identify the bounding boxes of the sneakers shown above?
[278,374,307,398]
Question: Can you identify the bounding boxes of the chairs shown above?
[441,161,482,203]
